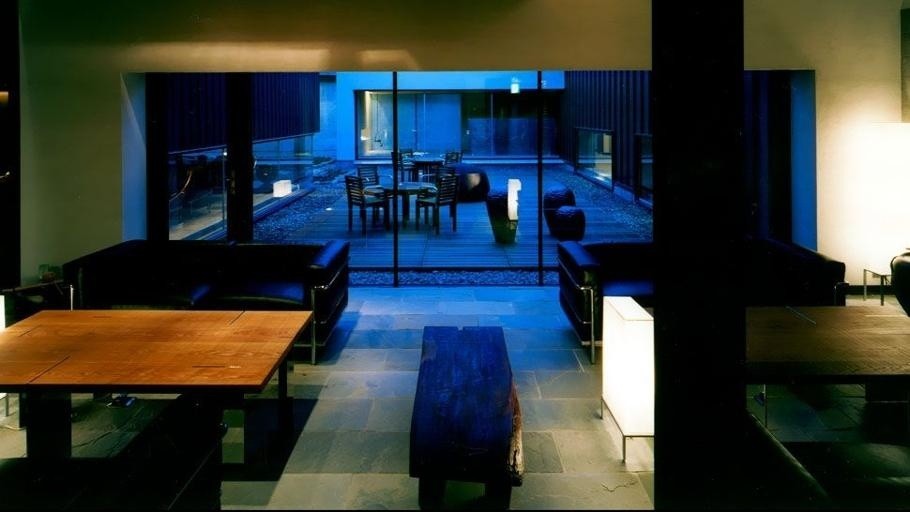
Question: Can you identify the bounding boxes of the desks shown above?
[0,309,316,510]
[639,306,910,508]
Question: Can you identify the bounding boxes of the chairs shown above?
[344,147,462,236]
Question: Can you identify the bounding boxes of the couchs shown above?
[556,231,848,365]
[61,238,351,363]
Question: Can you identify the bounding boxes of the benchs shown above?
[409,325,524,510]
